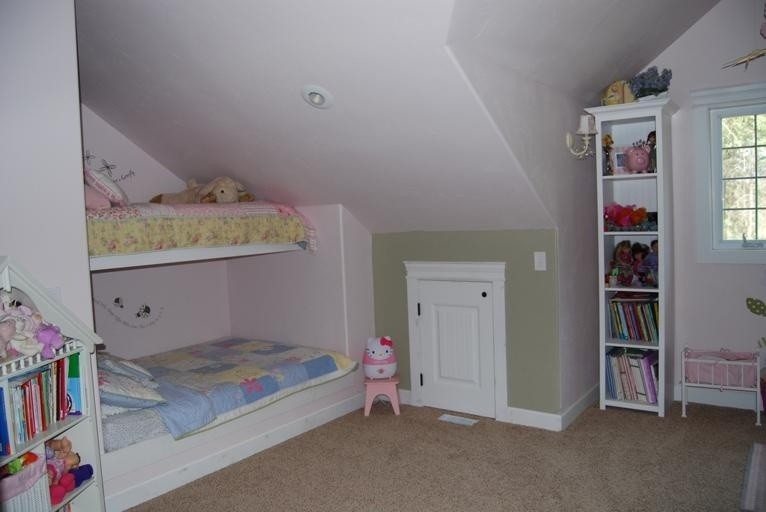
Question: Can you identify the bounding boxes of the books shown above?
[605,291,660,405]
[0,352,83,512]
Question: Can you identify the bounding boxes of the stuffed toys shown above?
[44,436,94,505]
[0,286,66,359]
[148,173,255,205]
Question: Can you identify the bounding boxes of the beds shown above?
[85,202,366,511]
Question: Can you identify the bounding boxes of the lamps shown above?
[565,114,598,160]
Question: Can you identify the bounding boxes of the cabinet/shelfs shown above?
[2,340,103,512]
[581,97,682,422]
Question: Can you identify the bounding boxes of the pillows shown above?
[93,350,166,412]
[84,165,124,211]
